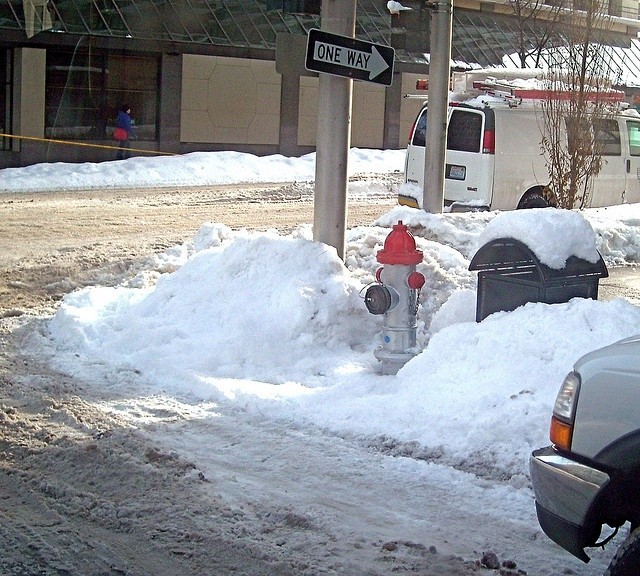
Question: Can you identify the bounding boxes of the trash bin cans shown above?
[469,237,609,323]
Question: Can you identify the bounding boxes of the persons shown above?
[113,106,131,160]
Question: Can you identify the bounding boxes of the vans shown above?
[397,98,640,211]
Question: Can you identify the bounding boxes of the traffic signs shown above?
[305,27,396,87]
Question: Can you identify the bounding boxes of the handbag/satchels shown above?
[114,115,130,142]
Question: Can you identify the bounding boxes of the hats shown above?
[121,103,130,112]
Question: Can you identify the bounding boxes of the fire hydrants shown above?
[359,220,425,376]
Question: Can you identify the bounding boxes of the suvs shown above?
[531,333,639,575]
